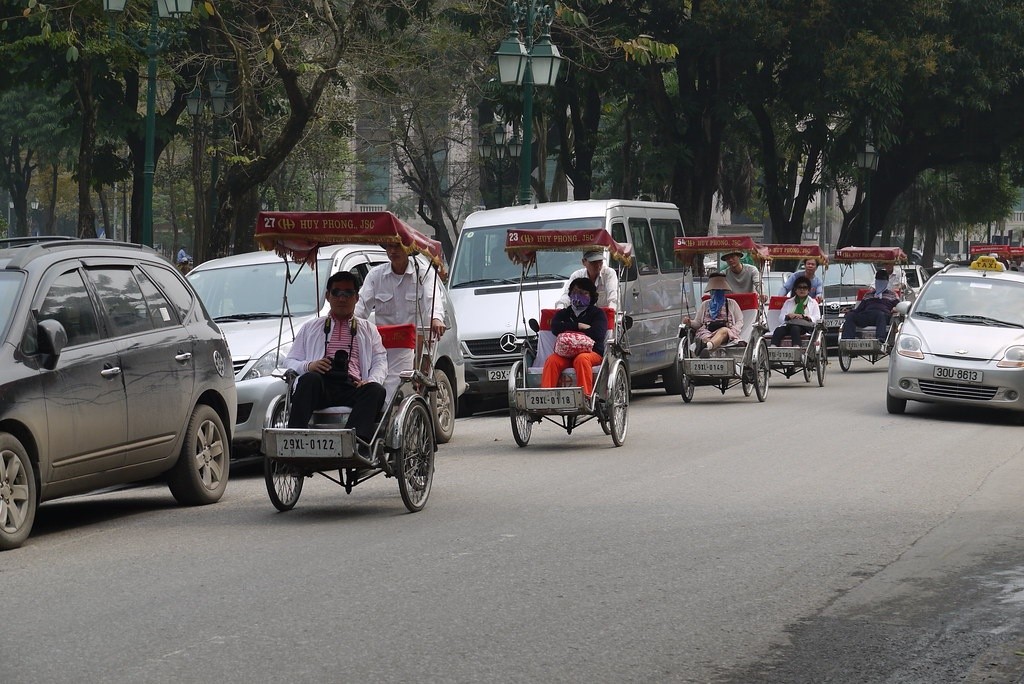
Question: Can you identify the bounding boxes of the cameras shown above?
[327,349,349,373]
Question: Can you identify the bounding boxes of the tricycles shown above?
[756,244,830,388]
[258,209,449,512]
[674,233,770,404]
[837,247,911,371]
[505,230,633,447]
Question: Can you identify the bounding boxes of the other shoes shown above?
[695,338,709,358]
[585,398,591,408]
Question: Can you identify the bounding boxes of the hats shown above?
[380,243,419,256]
[583,251,603,261]
[721,251,743,261]
[705,276,732,293]
[875,270,889,278]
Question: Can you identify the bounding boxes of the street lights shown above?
[104,0,193,248]
[494,0,563,208]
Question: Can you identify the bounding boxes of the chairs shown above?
[528,307,615,370]
[377,324,415,415]
[700,288,901,346]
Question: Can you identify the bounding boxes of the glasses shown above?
[331,289,357,297]
[796,286,808,290]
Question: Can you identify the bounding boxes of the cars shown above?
[912,249,944,269]
[181,244,466,458]
[695,261,929,345]
[886,256,1024,414]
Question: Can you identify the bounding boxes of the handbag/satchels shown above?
[553,330,595,358]
[706,320,726,332]
[785,315,814,333]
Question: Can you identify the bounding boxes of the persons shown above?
[541,277,607,401]
[352,244,447,411]
[841,270,900,343]
[719,251,768,304]
[284,271,389,457]
[988,253,1024,272]
[769,276,821,347]
[779,258,823,299]
[555,247,619,314]
[682,273,743,359]
[177,243,193,275]
[868,263,908,290]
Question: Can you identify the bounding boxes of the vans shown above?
[447,199,696,412]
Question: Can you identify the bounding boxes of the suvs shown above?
[0,235,238,549]
